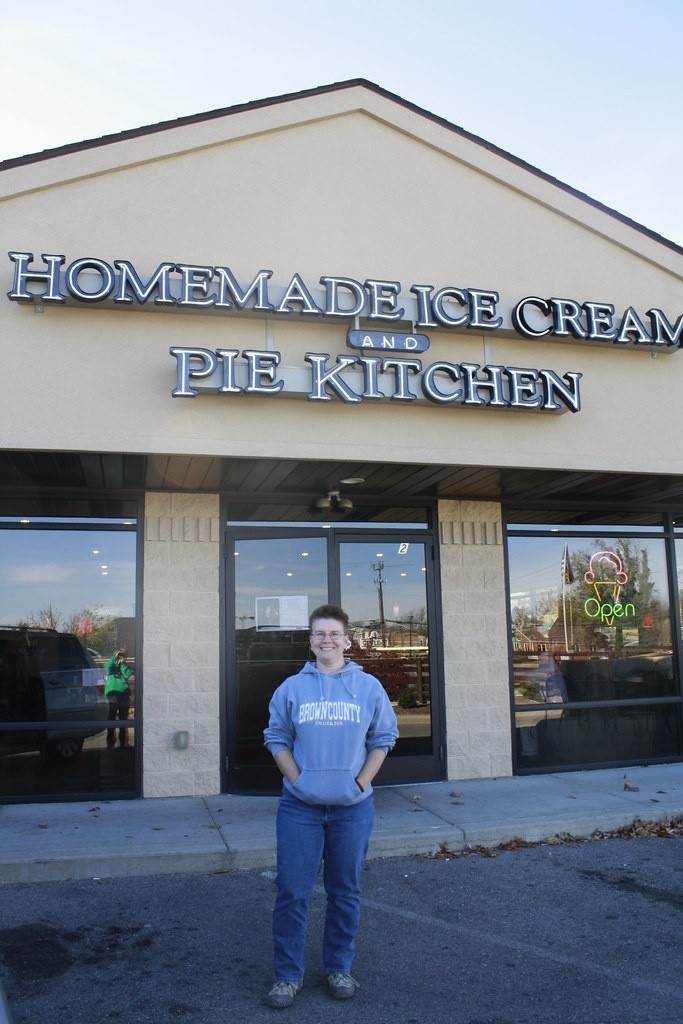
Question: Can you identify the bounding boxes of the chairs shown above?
[541,669,675,735]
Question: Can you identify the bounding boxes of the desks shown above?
[609,674,647,716]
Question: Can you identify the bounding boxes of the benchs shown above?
[536,713,657,765]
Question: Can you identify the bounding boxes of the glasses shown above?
[310,631,344,640]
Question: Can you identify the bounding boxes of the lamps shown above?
[314,491,354,511]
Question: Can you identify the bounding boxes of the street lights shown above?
[235,616,255,629]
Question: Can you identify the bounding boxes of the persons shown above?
[264,604,399,1007]
[104,645,134,750]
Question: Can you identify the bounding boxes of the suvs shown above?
[236,625,311,661]
[0,626,110,768]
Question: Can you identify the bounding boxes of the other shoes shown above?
[107,744,112,751]
[120,743,135,749]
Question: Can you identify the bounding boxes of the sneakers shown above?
[266,982,303,1008]
[327,973,360,999]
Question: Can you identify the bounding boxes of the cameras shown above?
[119,652,125,657]
